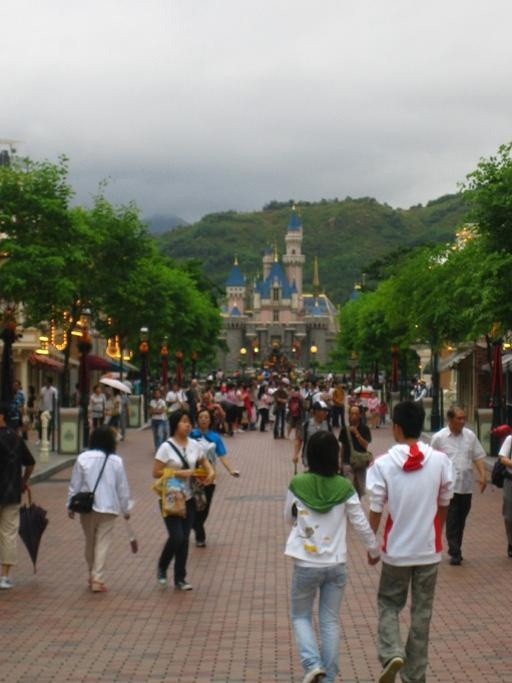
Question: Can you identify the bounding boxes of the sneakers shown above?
[88,577,108,592]
[379,656,405,682]
[447,548,464,565]
[0,576,13,588]
[157,568,167,584]
[175,579,192,590]
[195,539,205,547]
[303,665,325,683]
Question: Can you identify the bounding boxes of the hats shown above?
[313,400,332,410]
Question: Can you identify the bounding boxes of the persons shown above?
[0,401,35,588]
[431,407,487,564]
[338,405,371,500]
[365,400,457,683]
[188,411,240,546]
[283,432,380,683]
[65,425,133,592]
[292,401,332,470]
[8,380,25,435]
[26,384,36,424]
[36,376,57,445]
[152,411,215,591]
[498,435,512,557]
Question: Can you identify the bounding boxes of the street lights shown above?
[309,340,318,386]
[175,349,184,388]
[239,346,247,381]
[391,342,400,391]
[139,326,150,423]
[0,305,16,429]
[160,336,169,397]
[190,350,197,378]
[76,325,91,449]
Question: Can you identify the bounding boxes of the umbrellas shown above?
[19,485,49,575]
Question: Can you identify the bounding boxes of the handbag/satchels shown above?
[68,490,95,514]
[491,458,506,487]
[350,450,374,469]
[192,479,209,511]
